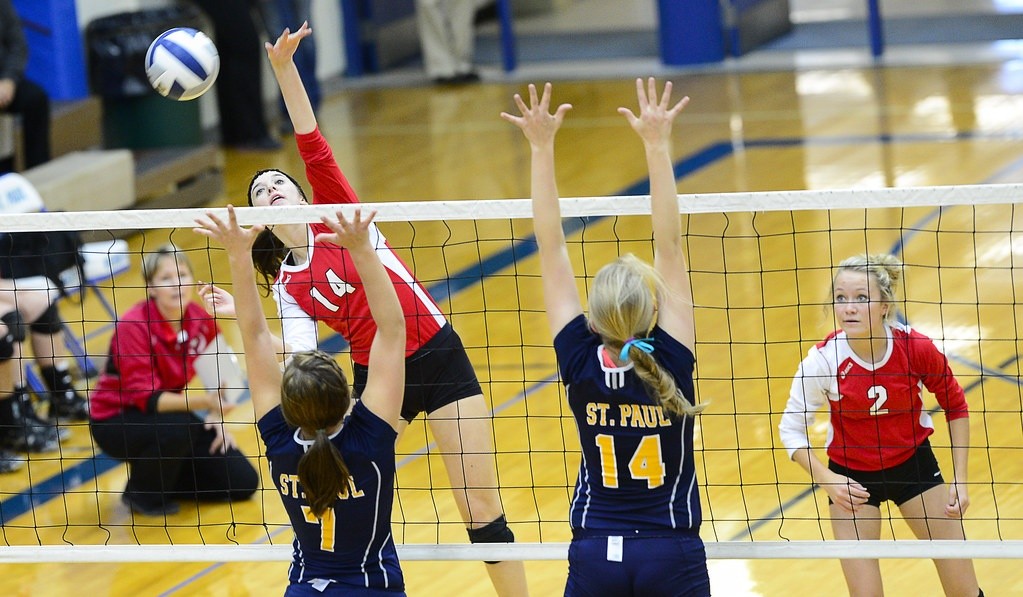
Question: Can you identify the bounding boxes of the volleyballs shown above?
[144,28,221,101]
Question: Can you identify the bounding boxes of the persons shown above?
[779,253,986,594]
[88,242,257,516]
[1,322,60,473]
[193,23,535,590]
[497,76,717,597]
[192,202,410,596]
[1,0,54,177]
[0,276,92,441]
[178,0,281,151]
[259,2,322,139]
[413,0,480,89]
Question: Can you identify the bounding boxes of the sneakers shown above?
[121,481,180,515]
[1,451,27,473]
[43,361,97,419]
[16,383,70,441]
[1,396,60,451]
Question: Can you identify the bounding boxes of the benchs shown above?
[358,13,421,70]
[79,146,218,250]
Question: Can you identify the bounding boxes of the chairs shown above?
[0,170,133,378]
